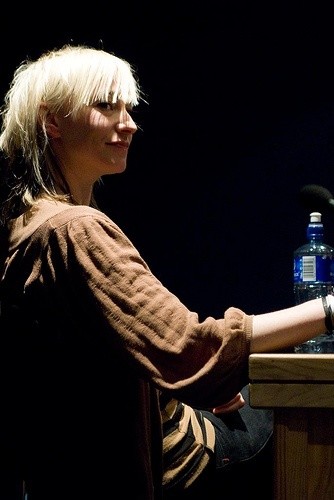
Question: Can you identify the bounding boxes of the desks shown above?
[248,354,334,500]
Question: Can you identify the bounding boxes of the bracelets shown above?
[320,295,334,337]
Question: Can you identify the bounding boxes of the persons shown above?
[0,46,334,500]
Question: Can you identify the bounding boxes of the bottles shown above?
[292,213,334,353]
